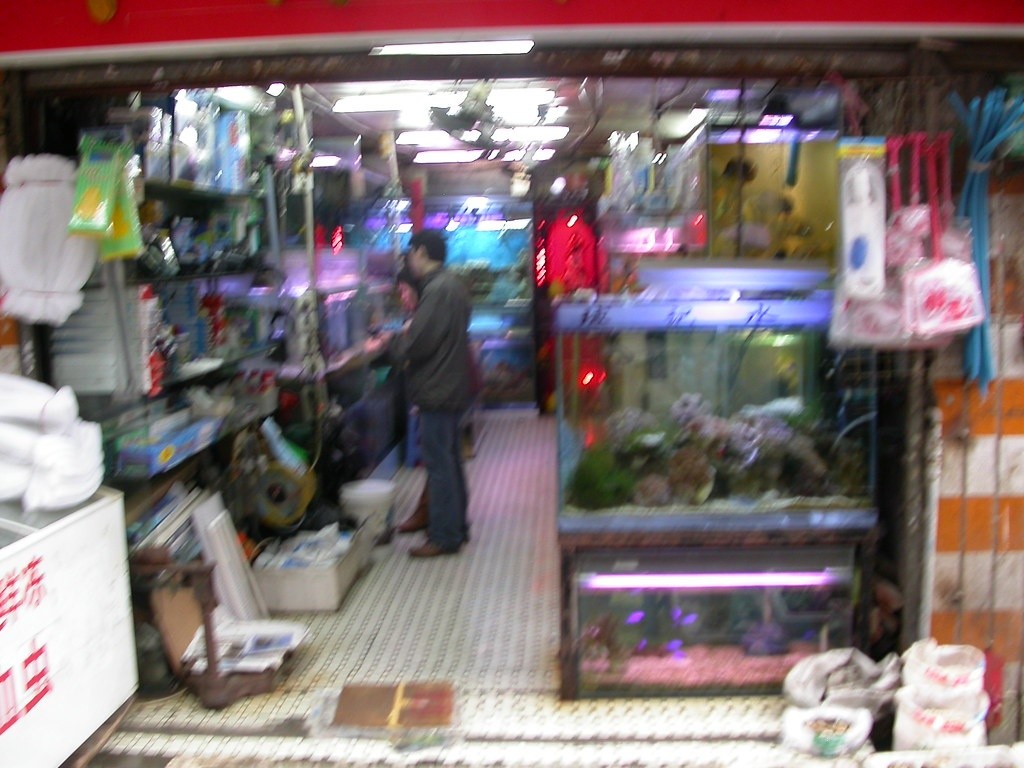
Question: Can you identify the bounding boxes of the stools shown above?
[408,408,424,468]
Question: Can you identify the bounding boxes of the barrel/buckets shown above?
[340,479,396,545]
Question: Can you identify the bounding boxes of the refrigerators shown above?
[0,486,140,768]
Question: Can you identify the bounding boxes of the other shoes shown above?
[410,541,445,556]
[398,506,426,533]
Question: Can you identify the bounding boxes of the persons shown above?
[387,229,474,559]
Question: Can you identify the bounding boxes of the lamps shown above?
[758,94,793,128]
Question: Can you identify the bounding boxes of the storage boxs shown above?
[350,194,535,309]
[475,309,541,408]
[696,124,842,262]
[559,530,873,701]
[257,530,362,613]
[551,271,881,538]
